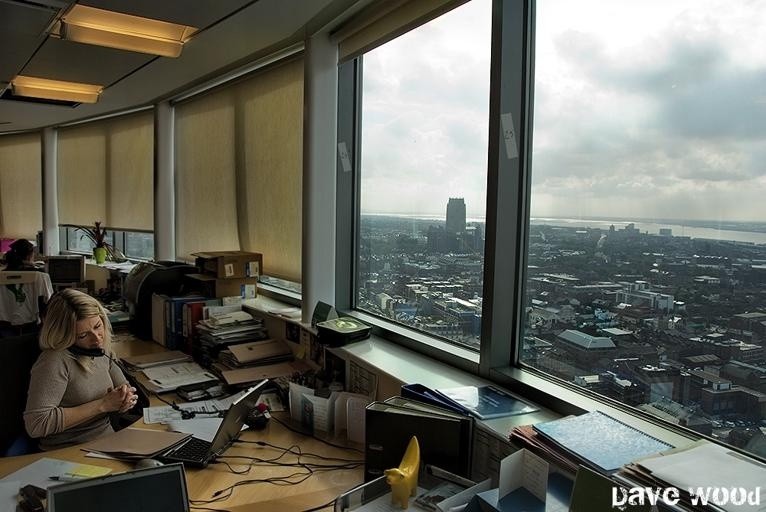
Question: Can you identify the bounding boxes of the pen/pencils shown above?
[49,477,83,481]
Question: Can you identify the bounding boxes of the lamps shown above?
[9,72,106,106]
[43,1,200,62]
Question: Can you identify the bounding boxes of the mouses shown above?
[135,459,165,472]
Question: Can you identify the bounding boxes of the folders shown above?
[363,384,476,505]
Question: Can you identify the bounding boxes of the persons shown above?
[1,238,44,273]
[20,287,138,453]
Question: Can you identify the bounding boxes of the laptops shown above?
[46,462,190,512]
[152,377,269,471]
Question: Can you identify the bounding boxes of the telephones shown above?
[66,344,105,357]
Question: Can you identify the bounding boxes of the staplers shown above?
[19,485,44,512]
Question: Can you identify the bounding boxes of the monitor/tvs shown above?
[43,255,86,284]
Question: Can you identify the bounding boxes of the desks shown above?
[1,321,381,511]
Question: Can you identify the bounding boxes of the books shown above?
[435,382,541,420]
[508,408,766,512]
[414,479,467,512]
[120,288,326,402]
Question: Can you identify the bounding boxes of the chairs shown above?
[0,271,47,356]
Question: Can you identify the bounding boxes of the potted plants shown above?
[73,213,110,268]
